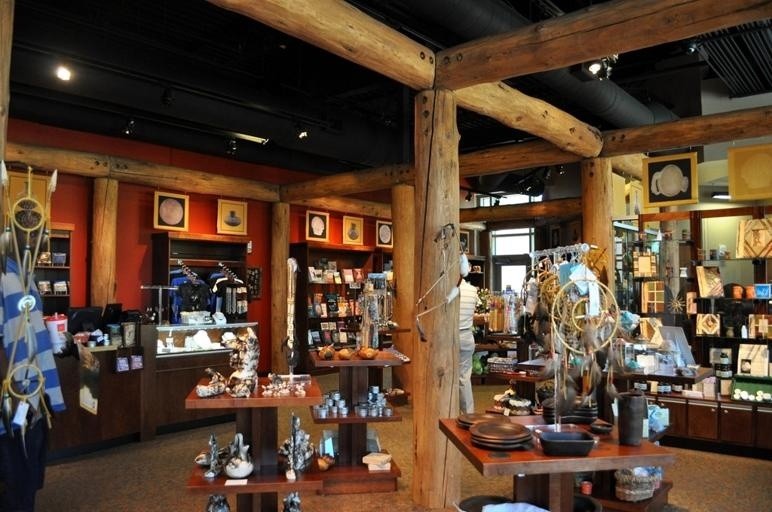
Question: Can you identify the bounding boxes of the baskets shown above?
[614,470,657,502]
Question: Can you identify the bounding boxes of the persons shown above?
[0,208,67,511]
[458,281,480,413]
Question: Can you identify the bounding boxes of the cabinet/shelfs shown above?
[24,224,73,324]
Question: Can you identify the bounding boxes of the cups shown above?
[731,285,754,298]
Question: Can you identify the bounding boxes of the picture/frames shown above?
[643,152,699,208]
[727,144,771,201]
[217,198,248,236]
[154,190,189,233]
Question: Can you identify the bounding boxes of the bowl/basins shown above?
[538,431,595,456]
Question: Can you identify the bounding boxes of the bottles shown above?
[225,210,241,226]
[707,245,731,259]
[725,326,735,338]
[741,325,747,338]
[632,376,683,394]
[313,385,393,420]
[715,352,733,379]
[347,223,359,240]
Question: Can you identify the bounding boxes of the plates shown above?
[456,412,534,447]
[159,197,183,225]
[541,396,598,425]
